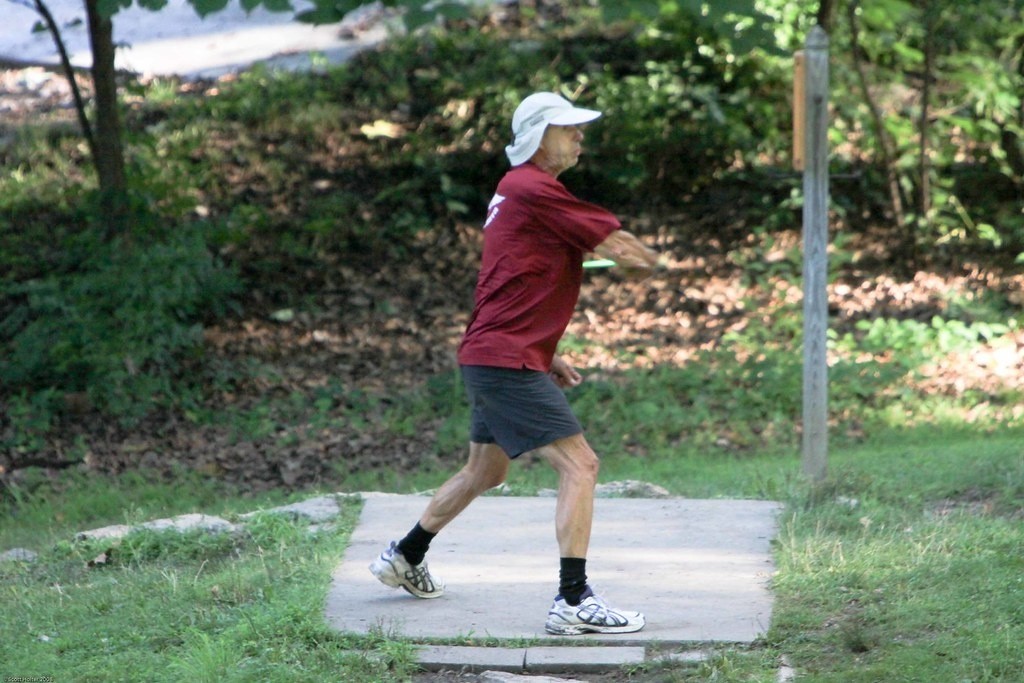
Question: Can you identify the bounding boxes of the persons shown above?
[370,91,663,634]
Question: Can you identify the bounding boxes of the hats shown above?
[505,92,602,166]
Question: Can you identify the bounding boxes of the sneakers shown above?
[545,587,646,635]
[369,542,445,597]
